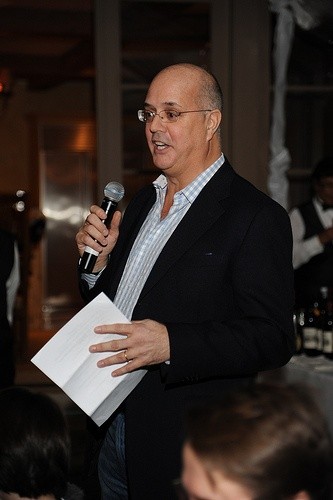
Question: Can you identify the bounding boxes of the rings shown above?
[123,350,129,361]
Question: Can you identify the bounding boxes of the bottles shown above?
[291,285,333,360]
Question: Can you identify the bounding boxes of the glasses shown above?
[137,109,212,123]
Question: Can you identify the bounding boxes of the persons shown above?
[76,61,299,500]
[1,387,71,500]
[177,380,332,500]
[1,192,29,390]
[287,156,333,309]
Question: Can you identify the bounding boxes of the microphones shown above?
[77,181,125,275]
